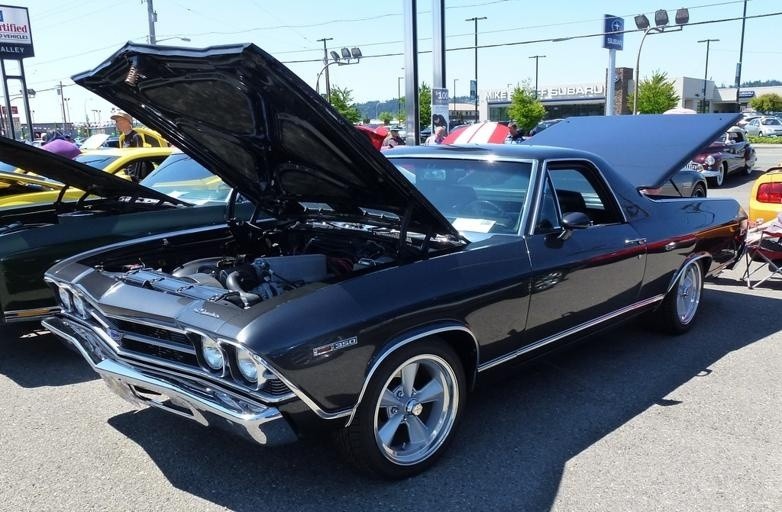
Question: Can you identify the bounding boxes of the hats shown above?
[388,125,403,132]
[109,110,133,126]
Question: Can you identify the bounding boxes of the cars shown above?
[36,36,752,480]
[520,119,565,141]
[638,169,708,198]
[734,106,782,138]
[746,166,781,230]
[684,126,760,190]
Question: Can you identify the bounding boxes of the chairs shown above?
[433,183,482,217]
[538,186,589,216]
[742,221,782,290]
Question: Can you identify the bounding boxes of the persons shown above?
[434,114,447,129]
[382,124,405,146]
[504,123,527,144]
[110,111,155,179]
[37,128,80,158]
[426,127,446,145]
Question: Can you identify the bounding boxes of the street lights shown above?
[0,87,37,114]
[316,38,333,104]
[396,76,405,119]
[375,102,386,117]
[526,53,546,100]
[696,39,719,112]
[314,47,362,99]
[633,8,689,115]
[91,107,119,124]
[465,16,488,121]
[452,78,460,115]
[63,97,72,122]
[147,36,192,48]
